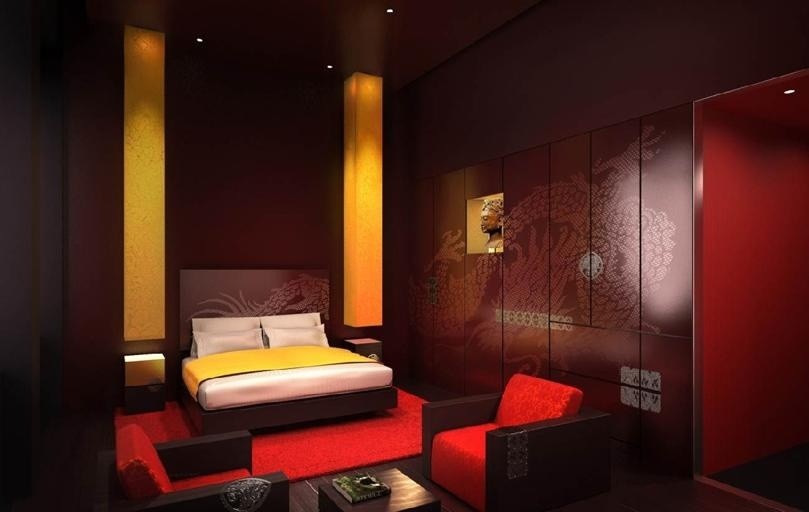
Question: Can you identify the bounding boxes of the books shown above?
[332,472,392,505]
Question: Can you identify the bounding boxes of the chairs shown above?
[108,423,289,512]
[421,373,612,512]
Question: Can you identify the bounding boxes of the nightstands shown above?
[344,338,382,359]
[121,353,166,414]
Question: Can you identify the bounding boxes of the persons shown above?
[478,197,504,254]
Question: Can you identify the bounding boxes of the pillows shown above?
[260,312,321,330]
[192,317,261,333]
[192,328,264,358]
[265,324,329,348]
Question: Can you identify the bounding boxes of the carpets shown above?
[114,385,430,484]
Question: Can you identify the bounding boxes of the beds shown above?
[176,267,397,437]
